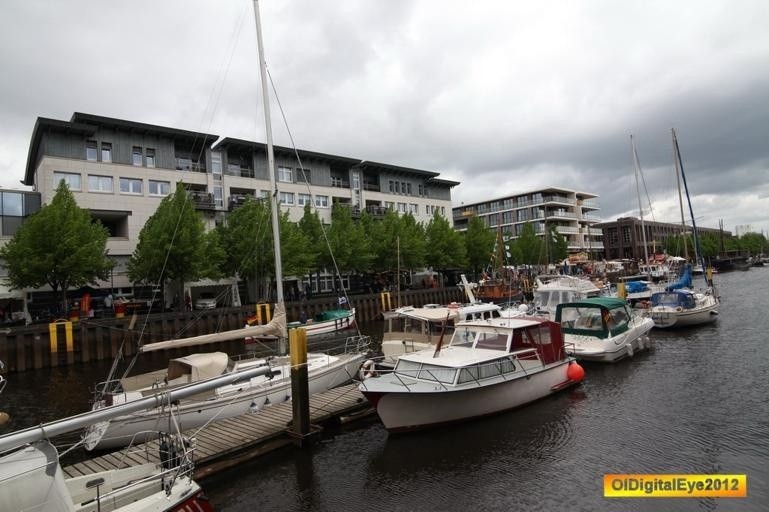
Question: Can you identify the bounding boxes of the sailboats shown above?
[84,0,373,451]
[456,200,611,304]
[601,128,722,330]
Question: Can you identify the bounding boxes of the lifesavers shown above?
[361,361,375,379]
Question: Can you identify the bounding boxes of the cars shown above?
[195,292,217,310]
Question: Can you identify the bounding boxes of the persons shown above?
[185,290,192,312]
[173,291,181,312]
[422,279,426,288]
[304,281,313,303]
[432,275,440,288]
[287,285,295,303]
[103,294,114,318]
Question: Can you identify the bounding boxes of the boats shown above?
[0,361,271,512]
[242,299,356,343]
